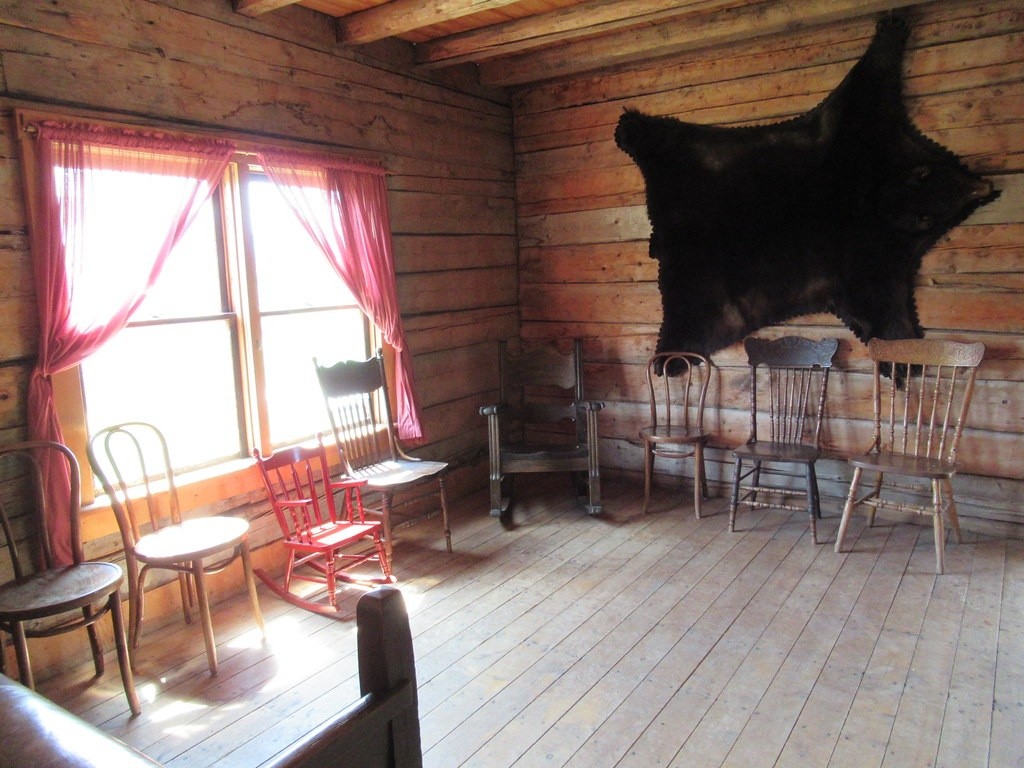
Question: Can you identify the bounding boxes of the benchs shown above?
[0,585,423,768]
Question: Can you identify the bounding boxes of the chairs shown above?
[252,431,397,616]
[0,441,141,716]
[641,353,715,520]
[312,348,454,574]
[479,335,607,518]
[85,422,267,676]
[729,337,840,547]
[833,337,986,574]
